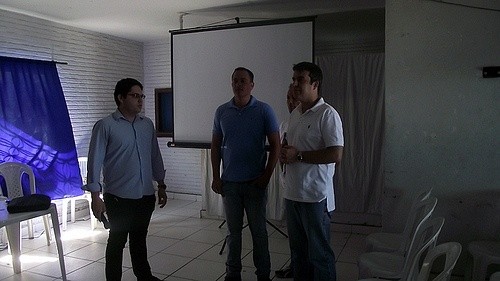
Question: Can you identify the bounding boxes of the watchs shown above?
[296,151,302,160]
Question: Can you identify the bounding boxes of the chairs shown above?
[467,238,500,281]
[357,185,462,281]
[0,157,103,281]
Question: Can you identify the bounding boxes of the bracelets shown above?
[158,184,166,190]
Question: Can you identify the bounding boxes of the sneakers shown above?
[276,265,293,277]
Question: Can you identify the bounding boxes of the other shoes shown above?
[143,277,161,281]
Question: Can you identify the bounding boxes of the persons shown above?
[272,61,345,281]
[275,83,302,278]
[81,78,167,281]
[211,67,281,281]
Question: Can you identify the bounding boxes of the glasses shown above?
[127,93,145,99]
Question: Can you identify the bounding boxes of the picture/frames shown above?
[155,88,174,138]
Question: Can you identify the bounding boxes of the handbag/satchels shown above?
[5,194,51,214]
